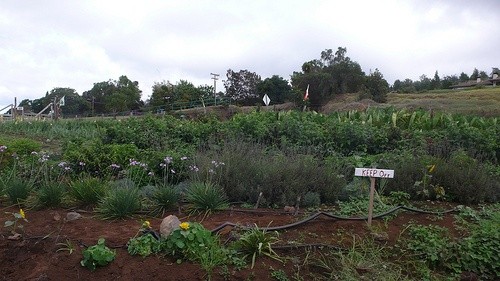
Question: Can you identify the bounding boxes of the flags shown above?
[60,96,64,106]
